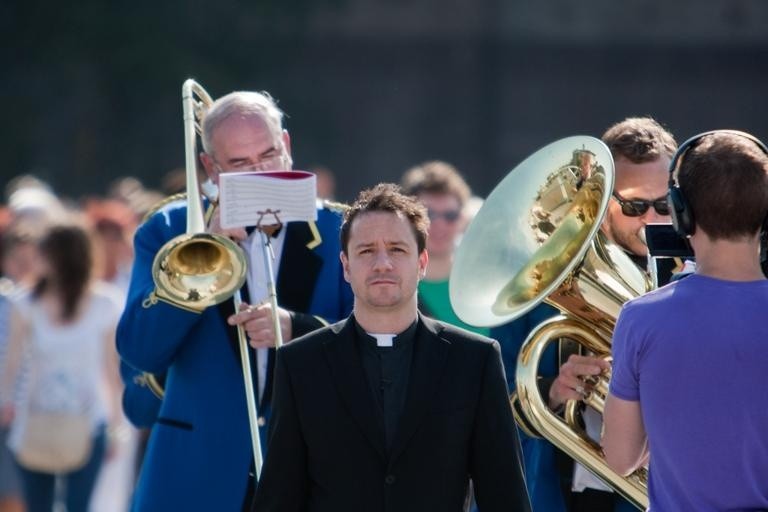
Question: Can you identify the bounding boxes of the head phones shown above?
[666,128,768,240]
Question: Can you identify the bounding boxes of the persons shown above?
[0,155,495,511]
[116,89,356,511]
[488,112,696,512]
[248,179,538,511]
[601,127,768,512]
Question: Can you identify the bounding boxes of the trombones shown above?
[151,78,263,486]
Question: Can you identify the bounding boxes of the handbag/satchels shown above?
[11,407,94,477]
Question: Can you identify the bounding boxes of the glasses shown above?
[426,204,461,222]
[213,151,289,175]
[613,191,672,218]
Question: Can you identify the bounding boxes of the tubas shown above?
[448,135,659,512]
